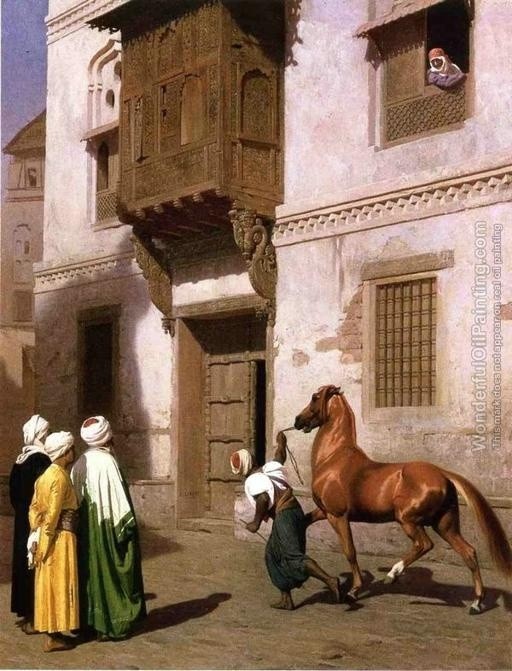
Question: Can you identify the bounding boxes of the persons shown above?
[25,430,85,655]
[424,46,466,89]
[7,413,53,636]
[68,412,148,644]
[230,428,345,613]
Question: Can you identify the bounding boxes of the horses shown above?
[294,385,512,616]
